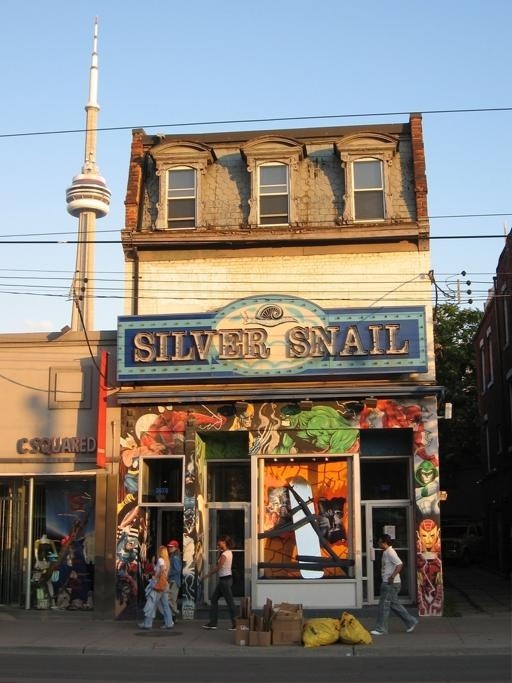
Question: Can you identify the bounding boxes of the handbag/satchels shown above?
[153,577,167,591]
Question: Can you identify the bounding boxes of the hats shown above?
[166,540,179,547]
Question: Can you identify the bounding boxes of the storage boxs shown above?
[234,603,304,646]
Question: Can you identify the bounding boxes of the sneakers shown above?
[136,622,152,629]
[370,630,384,636]
[160,622,174,629]
[227,626,236,631]
[202,622,217,630]
[406,620,419,633]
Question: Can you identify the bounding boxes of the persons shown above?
[370,534,418,636]
[138,544,174,631]
[161,539,183,624]
[200,534,240,631]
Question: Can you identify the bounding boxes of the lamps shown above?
[436,402,453,420]
[236,399,248,411]
[365,395,377,406]
[299,398,313,408]
